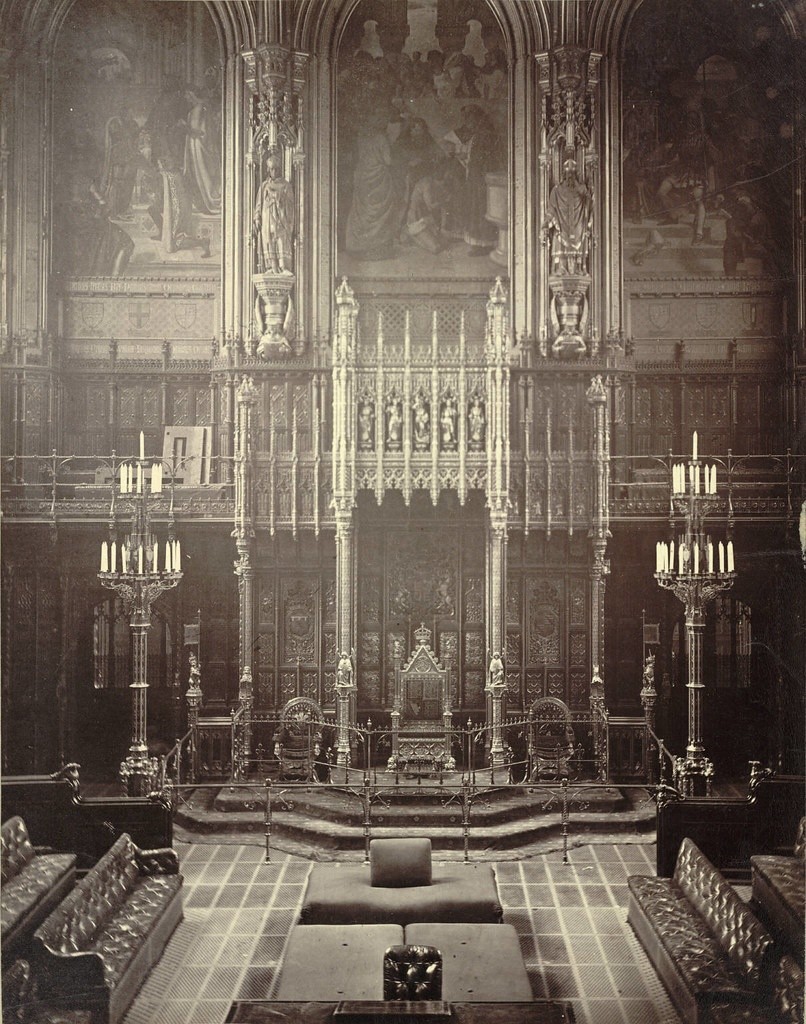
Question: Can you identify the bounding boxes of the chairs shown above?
[522,697,575,780]
[384,620,459,777]
[271,696,324,783]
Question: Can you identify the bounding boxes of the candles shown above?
[100,430,181,574]
[655,431,735,575]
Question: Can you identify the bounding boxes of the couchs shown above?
[383,945,443,1001]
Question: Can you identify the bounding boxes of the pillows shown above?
[370,838,432,888]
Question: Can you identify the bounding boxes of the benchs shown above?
[406,923,536,1002]
[301,865,503,922]
[2,958,92,1024]
[626,837,775,1024]
[0,815,77,985]
[705,950,806,1024]
[747,815,806,974]
[274,925,403,1002]
[33,832,185,1024]
